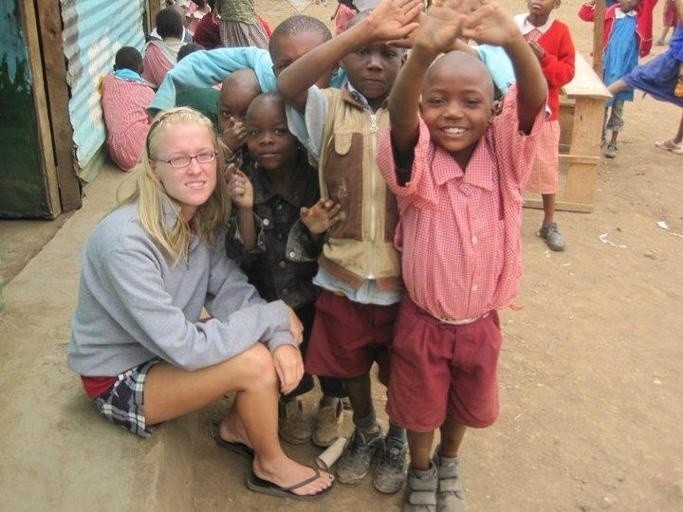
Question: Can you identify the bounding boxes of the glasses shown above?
[149,149,219,168]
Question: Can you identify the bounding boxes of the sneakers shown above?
[336,425,386,489]
[433,443,467,512]
[276,399,311,445]
[540,221,565,251]
[653,138,682,153]
[403,457,438,512]
[371,432,409,495]
[311,398,344,448]
[605,142,617,158]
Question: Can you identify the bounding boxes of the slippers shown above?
[246,465,333,502]
[214,433,255,460]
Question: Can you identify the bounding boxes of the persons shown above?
[211,0,270,49]
[214,69,262,137]
[142,8,188,92]
[192,21,220,50]
[177,44,206,64]
[375,0,549,512]
[606,0,683,156]
[224,93,350,448]
[274,1,407,495]
[65,104,337,499]
[268,15,341,89]
[578,0,656,160]
[653,0,683,46]
[101,47,156,172]
[512,0,576,253]
